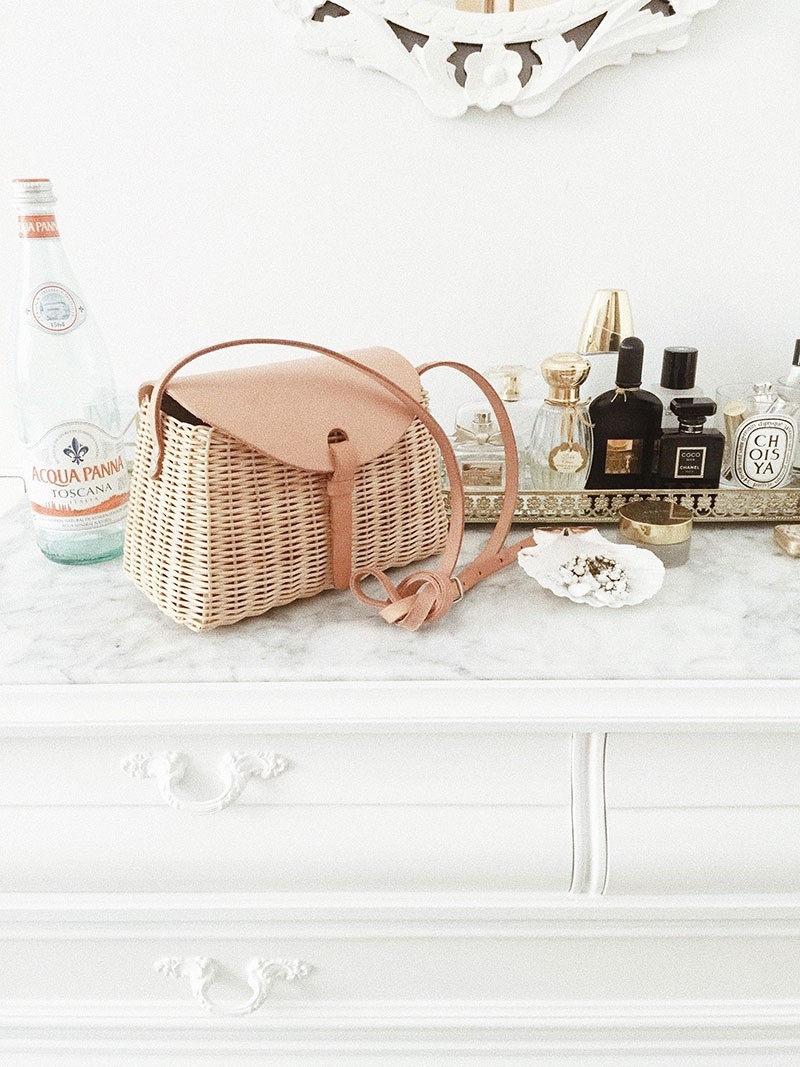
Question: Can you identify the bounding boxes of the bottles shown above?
[9,179,134,565]
[441,290,800,490]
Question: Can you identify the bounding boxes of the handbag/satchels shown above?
[123,338,537,634]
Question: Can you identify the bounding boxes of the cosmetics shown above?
[617,495,694,574]
[774,524,800,559]
[435,287,800,518]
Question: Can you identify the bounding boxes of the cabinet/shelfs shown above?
[0,522,799,1064]
